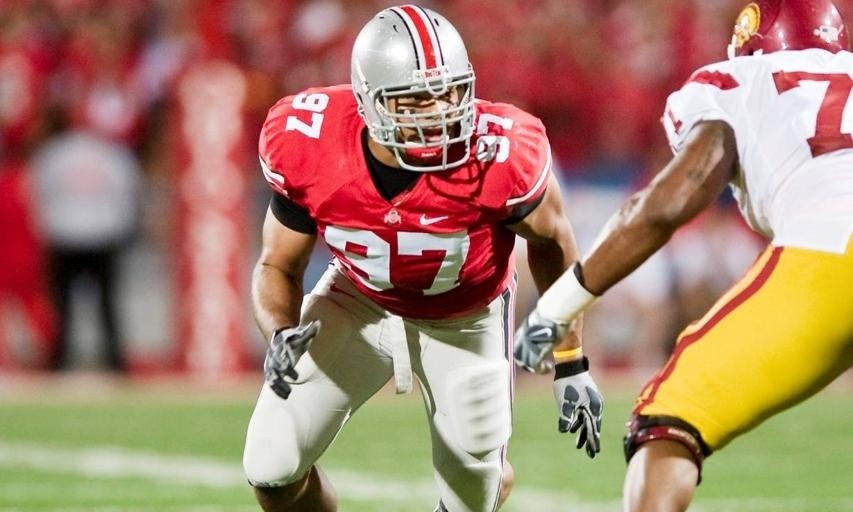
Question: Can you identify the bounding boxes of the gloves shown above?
[553,372,605,456]
[264,319,322,399]
[513,309,558,376]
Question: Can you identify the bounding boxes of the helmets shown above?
[729,0,850,53]
[352,5,475,173]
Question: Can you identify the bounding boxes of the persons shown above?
[0,0,771,372]
[242,7,602,512]
[514,0,853,512]
